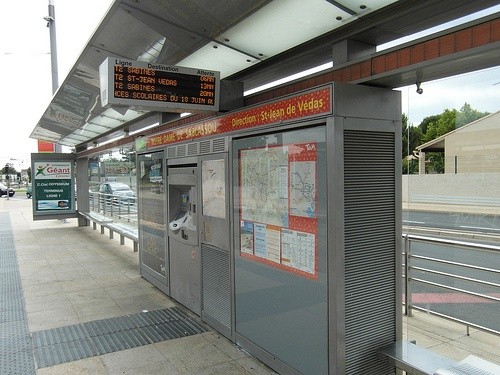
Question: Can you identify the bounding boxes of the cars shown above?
[26,186,32,199]
[0,182,15,198]
[99,182,136,207]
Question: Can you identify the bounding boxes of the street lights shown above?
[5,163,13,200]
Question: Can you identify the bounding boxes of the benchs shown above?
[80,210,113,234]
[104,221,140,252]
[377,339,460,374]
